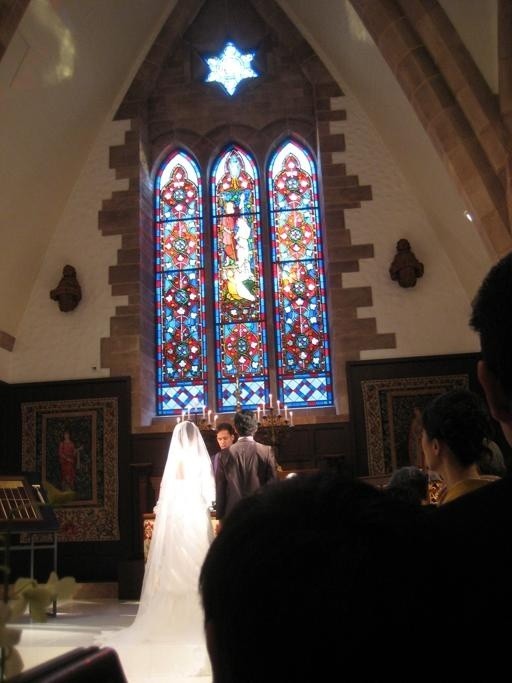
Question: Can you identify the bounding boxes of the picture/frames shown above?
[20,396,121,543]
[359,372,472,477]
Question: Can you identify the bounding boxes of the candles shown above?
[236,377,240,390]
[176,401,218,433]
[251,392,294,429]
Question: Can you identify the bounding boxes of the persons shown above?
[434,249,512,683]
[215,406,282,540]
[56,427,86,491]
[208,421,236,469]
[420,387,506,507]
[195,466,457,683]
[136,418,215,644]
[380,462,432,507]
